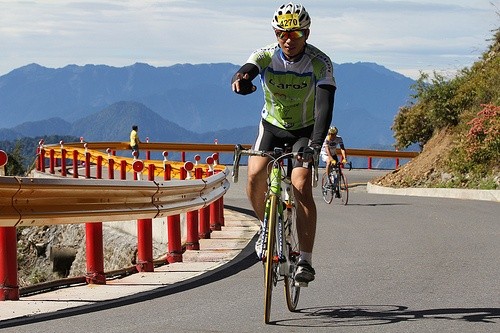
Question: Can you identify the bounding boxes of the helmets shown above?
[271,2,311,31]
[329,127,338,134]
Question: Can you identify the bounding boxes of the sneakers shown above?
[254,232,263,256]
[294,260,316,282]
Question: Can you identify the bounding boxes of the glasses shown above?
[274,29,306,39]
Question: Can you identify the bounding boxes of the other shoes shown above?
[334,193,340,198]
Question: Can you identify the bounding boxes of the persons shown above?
[130,125,141,159]
[231,2,336,282]
[321,126,347,198]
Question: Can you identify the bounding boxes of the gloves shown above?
[331,160,336,166]
[342,159,346,164]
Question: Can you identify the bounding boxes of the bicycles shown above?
[231,143,321,325]
[321,161,352,206]
